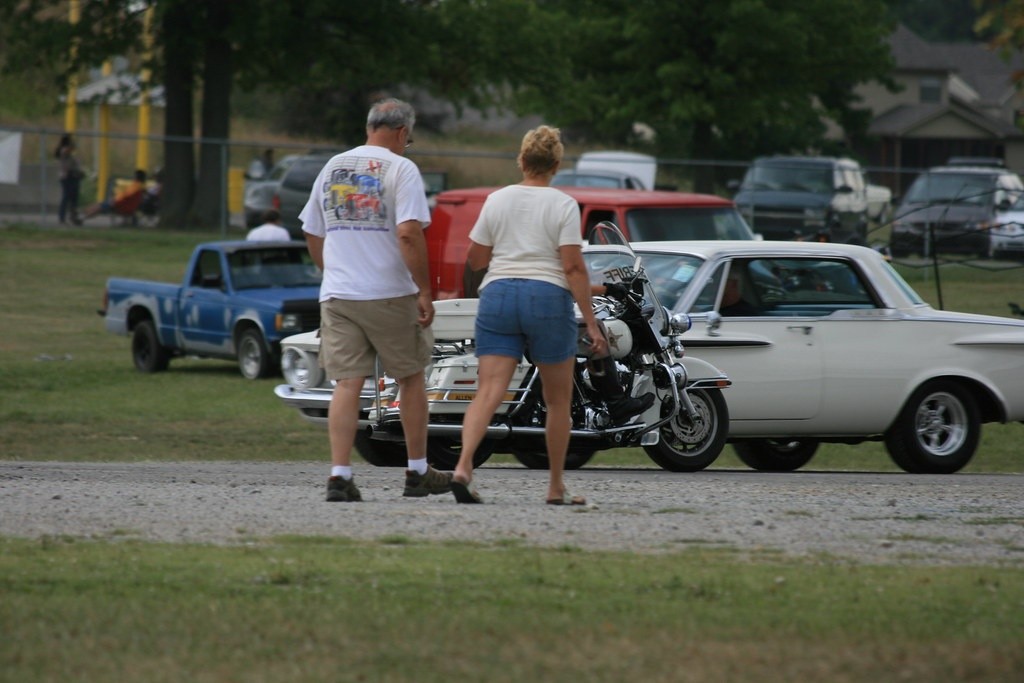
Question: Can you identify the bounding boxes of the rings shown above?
[597,346,601,349]
[594,344,597,346]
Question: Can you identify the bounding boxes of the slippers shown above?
[546,495,585,505]
[450,478,483,504]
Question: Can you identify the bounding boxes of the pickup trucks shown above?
[98,222,324,381]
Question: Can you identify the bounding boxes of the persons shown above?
[300,97,454,502]
[451,122,609,507]
[703,270,765,317]
[78,168,168,226]
[54,133,87,226]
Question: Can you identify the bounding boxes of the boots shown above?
[586,354,656,425]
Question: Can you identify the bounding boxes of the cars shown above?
[886,163,1024,259]
[272,240,1024,474]
[423,190,764,305]
[547,171,647,194]
[238,152,331,234]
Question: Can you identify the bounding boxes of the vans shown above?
[738,156,872,246]
[577,150,742,202]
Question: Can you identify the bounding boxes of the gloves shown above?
[602,282,629,301]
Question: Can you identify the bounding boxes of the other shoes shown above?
[70,211,84,225]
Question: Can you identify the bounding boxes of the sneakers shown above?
[326,474,361,502]
[402,464,453,497]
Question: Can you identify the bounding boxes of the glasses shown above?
[403,133,414,149]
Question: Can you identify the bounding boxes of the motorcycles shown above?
[364,219,733,474]
